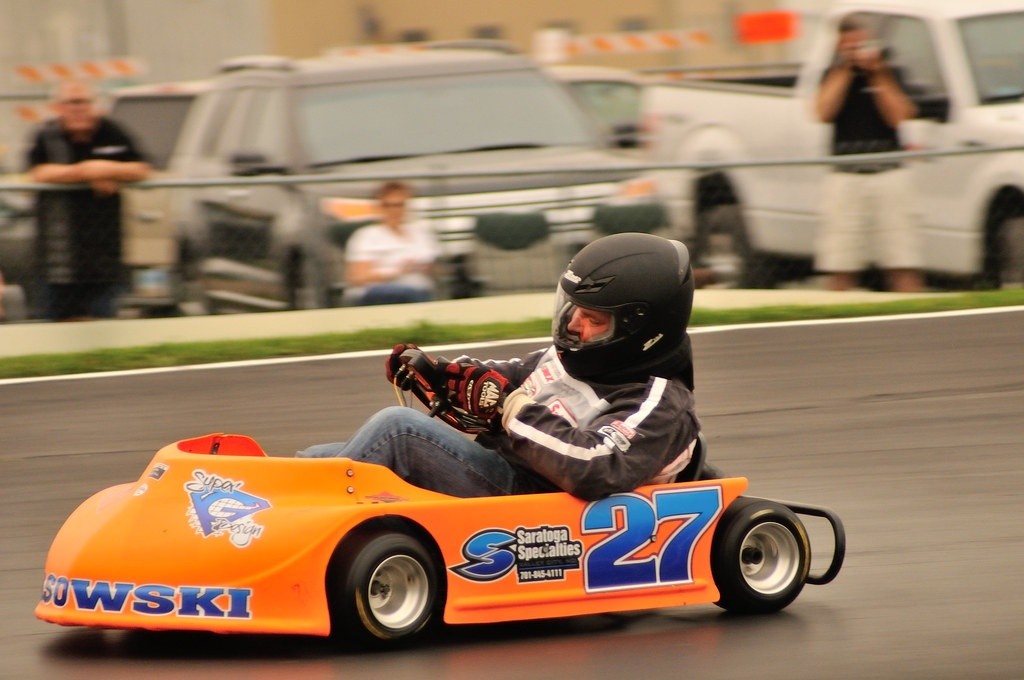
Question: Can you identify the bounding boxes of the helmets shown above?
[551,232,694,385]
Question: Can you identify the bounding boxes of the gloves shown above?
[386,343,442,393]
[438,355,517,434]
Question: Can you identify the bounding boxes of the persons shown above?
[297,232,701,500]
[814,13,923,293]
[342,183,439,304]
[29,82,155,320]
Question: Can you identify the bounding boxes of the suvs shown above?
[2,79,200,305]
[164,39,679,312]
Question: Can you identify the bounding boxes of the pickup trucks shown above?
[650,0,1024,296]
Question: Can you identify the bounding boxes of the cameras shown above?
[849,41,882,67]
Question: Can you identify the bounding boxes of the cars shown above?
[547,63,646,158]
